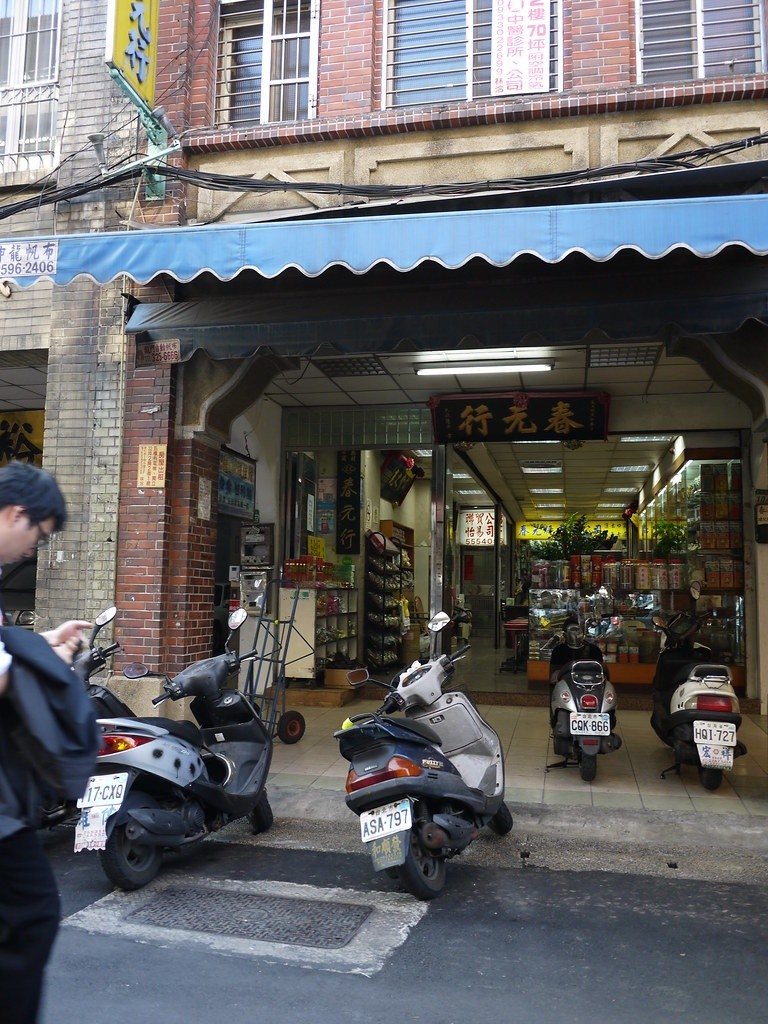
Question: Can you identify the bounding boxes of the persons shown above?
[530,591,570,610]
[632,592,641,607]
[0,461,96,1024]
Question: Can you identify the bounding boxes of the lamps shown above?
[412,357,556,377]
[149,105,180,146]
[86,133,108,177]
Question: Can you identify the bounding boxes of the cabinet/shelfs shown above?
[528,434,747,696]
[233,588,359,697]
[379,519,415,613]
[367,531,402,675]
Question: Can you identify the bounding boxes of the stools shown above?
[499,618,528,674]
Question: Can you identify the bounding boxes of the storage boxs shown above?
[323,668,358,689]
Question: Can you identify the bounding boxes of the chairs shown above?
[415,596,431,628]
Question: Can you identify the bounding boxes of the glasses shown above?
[25,512,51,550]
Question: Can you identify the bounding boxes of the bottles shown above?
[531,554,686,589]
[640,631,661,664]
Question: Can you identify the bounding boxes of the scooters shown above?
[333,611,512,900]
[73,608,273,890]
[37,607,138,829]
[539,617,622,781]
[649,581,748,791]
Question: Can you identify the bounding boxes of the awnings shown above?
[0,192,768,288]
